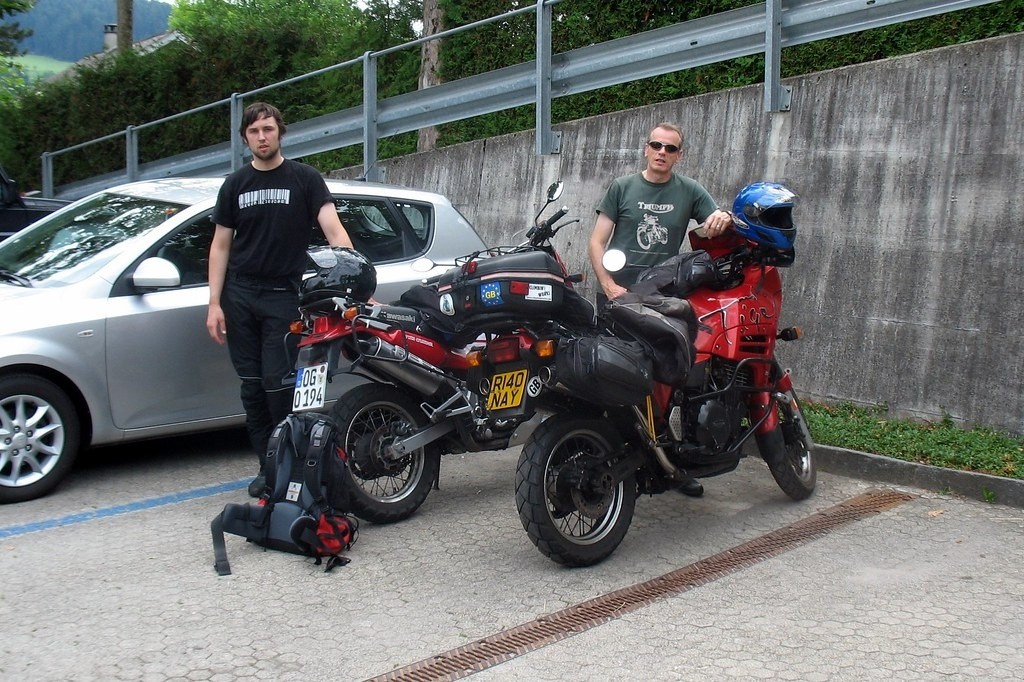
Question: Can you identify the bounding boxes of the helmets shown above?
[731,182,797,250]
[297,244,377,310]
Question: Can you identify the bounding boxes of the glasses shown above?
[648,142,679,153]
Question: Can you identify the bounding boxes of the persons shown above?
[589,121,731,496]
[206,102,381,500]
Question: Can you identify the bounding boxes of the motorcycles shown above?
[283,181,584,523]
[515,222,817,566]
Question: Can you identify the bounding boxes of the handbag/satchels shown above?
[636,249,718,297]
[573,335,652,407]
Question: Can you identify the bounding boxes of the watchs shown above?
[721,210,732,218]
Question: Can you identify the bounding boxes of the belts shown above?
[225,269,299,291]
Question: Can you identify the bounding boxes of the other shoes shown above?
[248,474,265,498]
[679,478,703,496]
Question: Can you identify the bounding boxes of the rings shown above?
[717,225,721,227]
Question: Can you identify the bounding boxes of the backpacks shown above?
[211,412,359,576]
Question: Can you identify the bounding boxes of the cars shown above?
[0,166,496,503]
